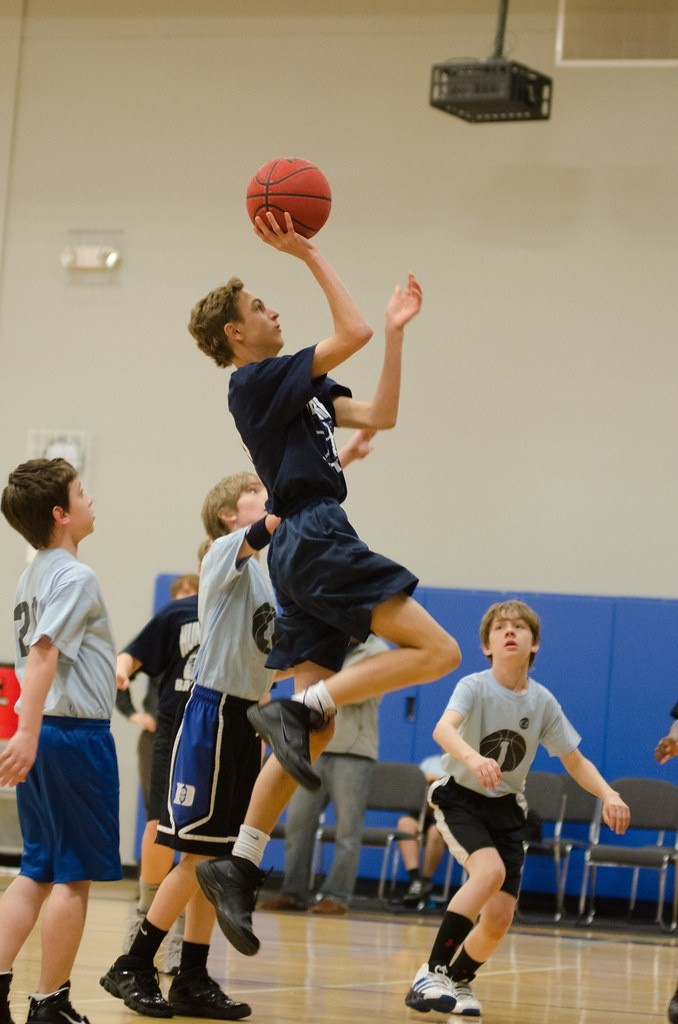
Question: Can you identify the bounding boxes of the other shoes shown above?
[310,891,349,916]
[256,891,309,912]
[122,906,147,954]
[402,877,434,911]
[162,935,187,975]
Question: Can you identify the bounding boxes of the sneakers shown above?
[169,967,251,1020]
[246,695,330,793]
[405,963,457,1013]
[0,969,18,1024]
[195,859,274,956]
[25,980,91,1024]
[449,973,481,1016]
[100,955,176,1018]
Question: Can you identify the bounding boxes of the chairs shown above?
[271,760,678,934]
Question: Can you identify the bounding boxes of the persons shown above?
[188,210,423,956]
[1,451,123,1024]
[403,600,632,1017]
[655,704,678,1024]
[100,472,449,1018]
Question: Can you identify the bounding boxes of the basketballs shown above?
[244,157,332,241]
